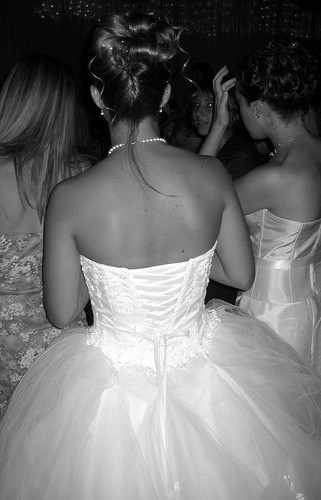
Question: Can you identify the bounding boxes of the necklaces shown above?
[107,137,168,156]
[268,132,306,158]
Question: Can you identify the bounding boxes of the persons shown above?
[197,39,321,375]
[190,73,249,181]
[0,9,321,500]
[0,53,94,415]
